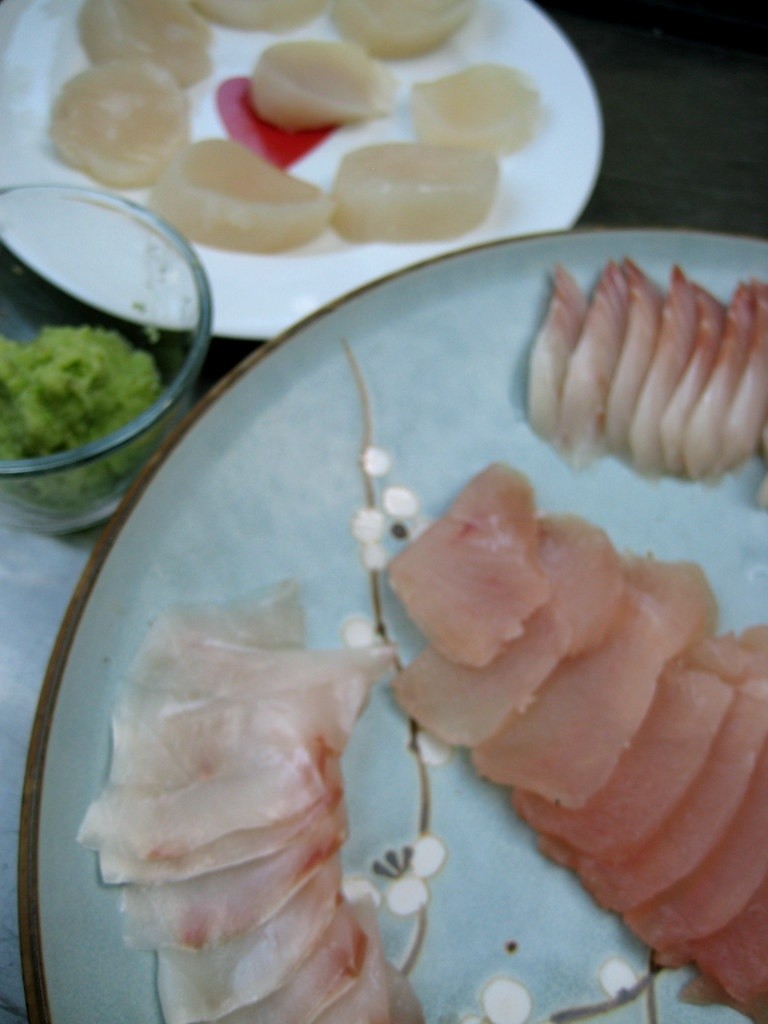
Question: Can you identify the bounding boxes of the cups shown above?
[0,185,213,536]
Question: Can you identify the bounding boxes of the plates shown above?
[0,0,603,340]
[16,224,768,1024]
